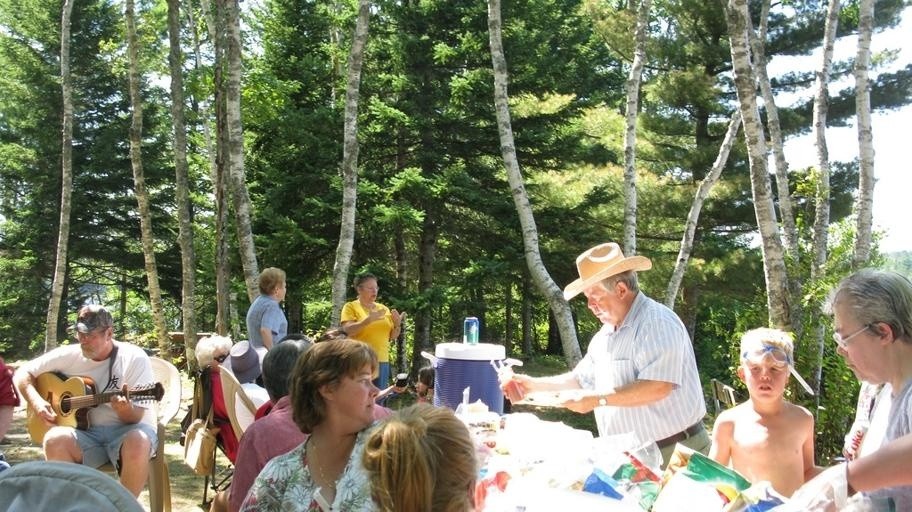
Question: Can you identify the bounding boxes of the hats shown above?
[67,304,110,332]
[219,340,270,382]
[193,335,231,369]
[564,243,653,302]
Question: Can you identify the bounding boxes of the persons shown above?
[195,332,436,434]
[11,305,165,499]
[208,339,394,512]
[340,272,405,391]
[363,403,478,512]
[498,243,711,467]
[239,342,387,512]
[707,327,830,499]
[0,358,20,443]
[793,268,912,512]
[246,267,289,347]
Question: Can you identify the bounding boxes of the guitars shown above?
[28,372,164,443]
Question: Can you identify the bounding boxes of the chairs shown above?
[1,456,148,512]
[711,378,735,423]
[95,357,182,512]
[191,364,257,506]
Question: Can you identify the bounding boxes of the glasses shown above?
[831,326,869,350]
[357,286,380,291]
[740,345,790,367]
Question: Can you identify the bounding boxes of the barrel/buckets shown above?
[433,341,508,416]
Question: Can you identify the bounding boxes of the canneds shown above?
[463,317,479,345]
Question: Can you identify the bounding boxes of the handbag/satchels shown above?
[184,417,218,475]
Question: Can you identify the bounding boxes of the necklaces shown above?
[309,438,336,489]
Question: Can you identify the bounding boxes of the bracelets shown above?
[393,326,401,331]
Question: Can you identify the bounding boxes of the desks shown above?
[462,413,788,512]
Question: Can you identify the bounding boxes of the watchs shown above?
[598,392,606,407]
